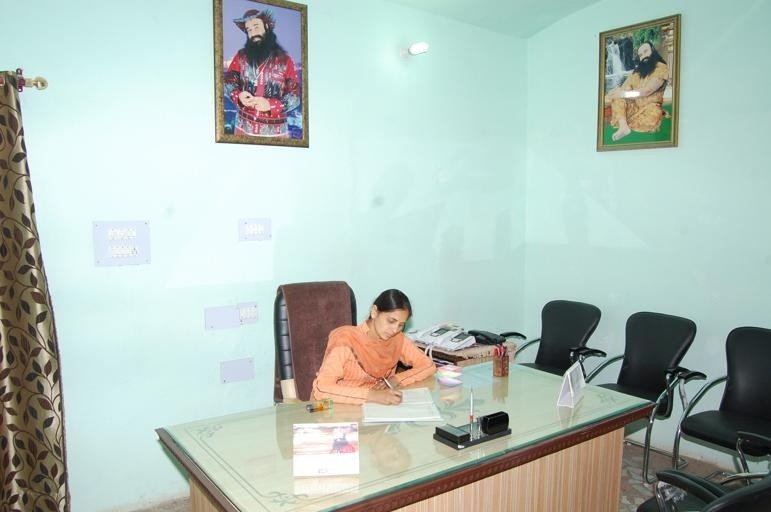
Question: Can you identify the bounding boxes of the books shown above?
[362,386,445,422]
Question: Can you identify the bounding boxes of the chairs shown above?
[500,300,601,376]
[673,326,771,486]
[579,311,706,485]
[273,281,357,403]
[637,468,771,511]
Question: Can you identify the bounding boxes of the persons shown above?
[605,40,671,145]
[308,289,437,404]
[227,7,303,139]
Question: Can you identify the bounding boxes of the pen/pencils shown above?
[470,386,473,441]
[383,376,394,390]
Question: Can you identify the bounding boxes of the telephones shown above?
[416,323,507,351]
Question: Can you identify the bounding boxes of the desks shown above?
[154,357,658,511]
[397,339,517,374]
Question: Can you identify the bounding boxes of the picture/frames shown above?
[597,13,682,153]
[213,0,310,148]
